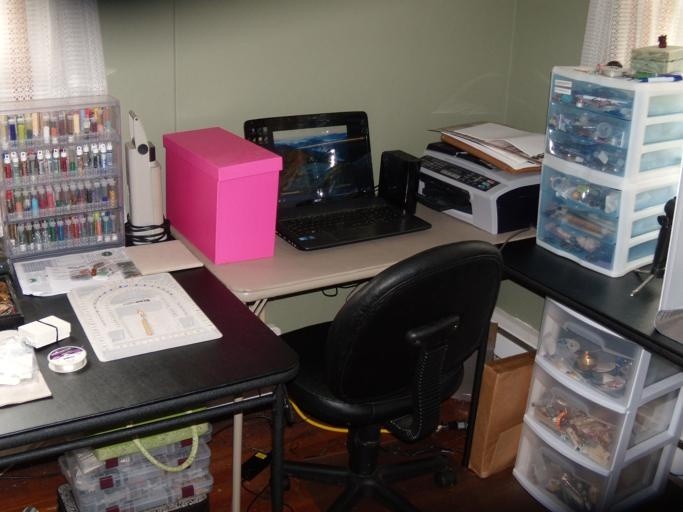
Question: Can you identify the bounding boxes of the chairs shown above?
[268,240,505,512]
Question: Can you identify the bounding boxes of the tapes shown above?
[602,66,624,77]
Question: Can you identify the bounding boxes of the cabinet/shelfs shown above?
[0,92,126,261]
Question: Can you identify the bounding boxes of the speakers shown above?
[379,150,421,217]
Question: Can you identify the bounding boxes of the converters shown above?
[241,451,271,481]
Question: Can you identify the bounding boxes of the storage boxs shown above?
[162,126,285,267]
[535,63,683,278]
[512,297,683,512]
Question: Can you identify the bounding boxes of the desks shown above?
[0,234,303,512]
[462,236,682,468]
[167,202,538,512]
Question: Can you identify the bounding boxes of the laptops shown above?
[245,112,432,252]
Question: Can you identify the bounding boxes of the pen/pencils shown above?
[639,76,682,83]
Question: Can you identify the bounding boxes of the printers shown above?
[418,141,541,235]
